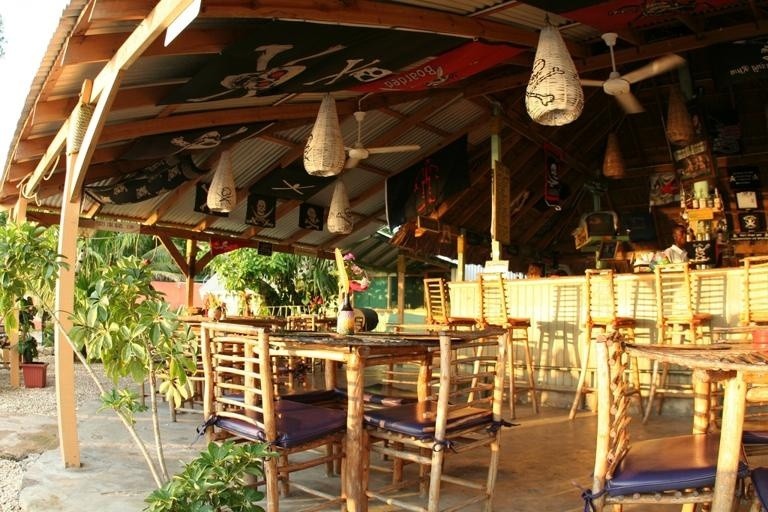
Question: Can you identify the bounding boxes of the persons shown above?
[663,225,695,270]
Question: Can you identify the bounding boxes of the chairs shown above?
[590,330,768,512]
[476,273,540,419]
[134,309,508,512]
[424,278,478,398]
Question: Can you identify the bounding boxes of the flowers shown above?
[296,258,324,313]
[332,250,369,303]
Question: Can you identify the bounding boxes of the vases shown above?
[336,294,355,336]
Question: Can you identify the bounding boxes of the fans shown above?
[579,33,686,112]
[346,111,420,169]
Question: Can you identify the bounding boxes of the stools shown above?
[643,261,712,421]
[569,268,645,421]
[739,254,768,330]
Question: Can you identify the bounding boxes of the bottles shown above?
[679,186,721,242]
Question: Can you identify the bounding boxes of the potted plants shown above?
[15,298,49,387]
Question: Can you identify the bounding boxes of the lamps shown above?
[525,14,585,126]
[206,147,237,212]
[302,90,346,176]
[603,131,626,179]
[666,87,694,147]
[327,181,354,235]
